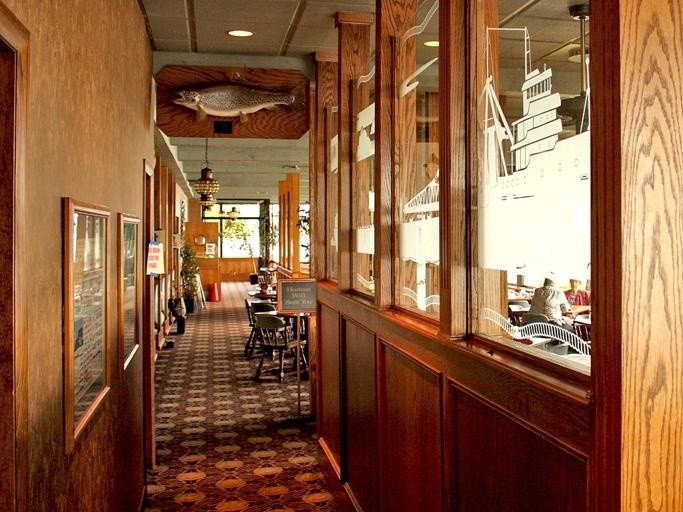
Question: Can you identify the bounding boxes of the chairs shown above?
[242,288,310,384]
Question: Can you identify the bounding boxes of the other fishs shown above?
[170,81,306,124]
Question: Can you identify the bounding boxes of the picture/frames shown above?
[114,211,144,378]
[59,195,115,458]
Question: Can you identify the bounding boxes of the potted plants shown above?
[179,245,200,314]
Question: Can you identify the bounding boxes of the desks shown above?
[508,289,593,355]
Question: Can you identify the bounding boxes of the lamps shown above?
[198,196,217,211]
[226,206,242,218]
[194,138,220,196]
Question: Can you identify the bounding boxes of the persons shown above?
[558,277,591,319]
[530,277,574,333]
